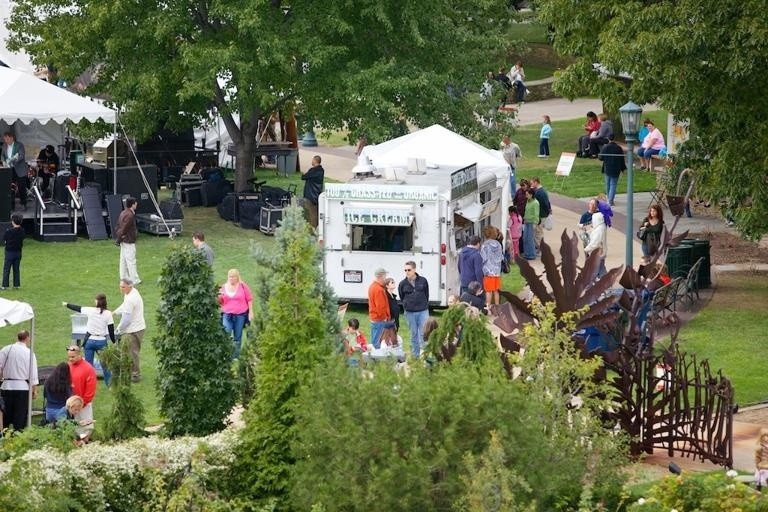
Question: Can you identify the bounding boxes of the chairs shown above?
[644,173,669,209]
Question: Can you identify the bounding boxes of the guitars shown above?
[3,153,18,167]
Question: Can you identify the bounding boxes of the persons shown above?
[582,198,668,289]
[0,131,30,207]
[578,110,612,158]
[301,153,324,233]
[754,427,768,491]
[335,261,443,371]
[215,268,256,356]
[499,135,522,200]
[508,176,551,259]
[537,115,552,158]
[190,232,214,273]
[598,134,626,205]
[480,63,530,103]
[636,117,665,172]
[37,144,62,195]
[114,197,145,288]
[0,211,23,295]
[449,226,504,345]
[0,279,147,445]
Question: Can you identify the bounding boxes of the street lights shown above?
[616,95,644,295]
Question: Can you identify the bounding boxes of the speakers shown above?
[185,186,201,207]
[0,167,15,222]
[218,185,291,230]
[176,180,207,203]
[76,162,106,208]
[181,174,203,182]
[158,201,184,219]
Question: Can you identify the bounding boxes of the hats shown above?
[45,145,54,154]
[375,266,389,276]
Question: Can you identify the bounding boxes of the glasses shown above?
[404,267,414,272]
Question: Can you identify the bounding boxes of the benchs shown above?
[608,139,666,173]
[645,255,705,333]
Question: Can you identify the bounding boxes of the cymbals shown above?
[30,159,44,162]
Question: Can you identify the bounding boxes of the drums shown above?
[42,163,56,174]
[28,164,36,179]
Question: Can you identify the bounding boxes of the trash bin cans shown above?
[664,239,710,292]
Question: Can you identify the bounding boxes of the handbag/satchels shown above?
[636,217,649,242]
[242,310,250,329]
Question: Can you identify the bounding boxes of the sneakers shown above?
[537,154,550,159]
[1,287,20,290]
